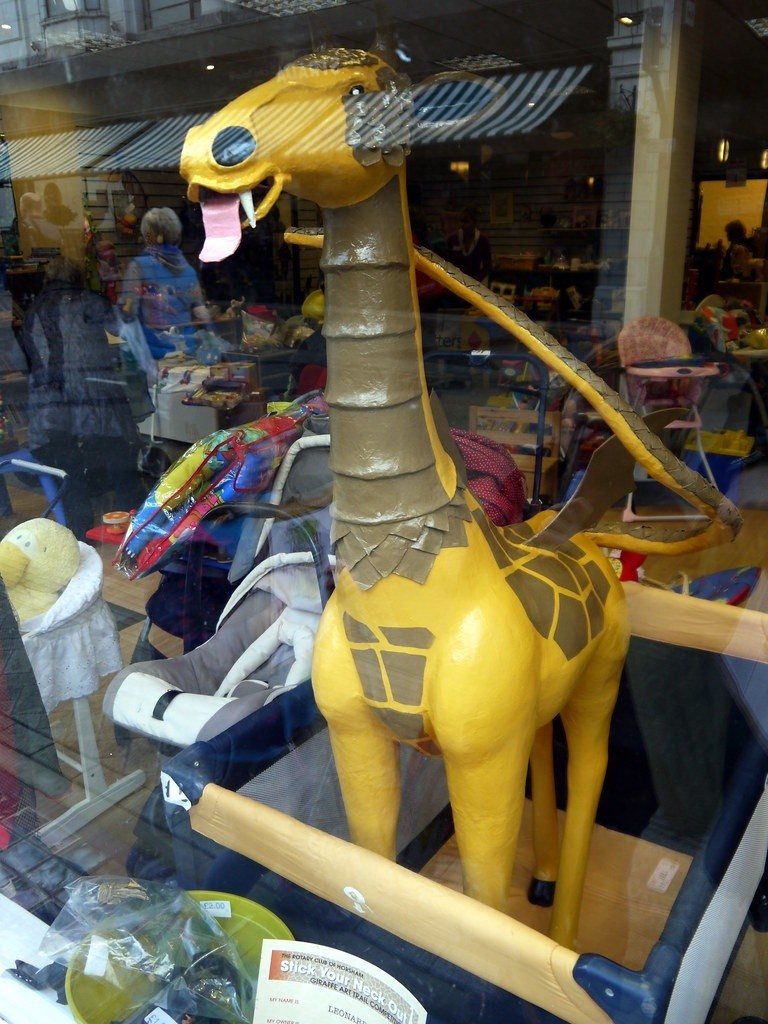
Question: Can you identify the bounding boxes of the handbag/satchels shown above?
[111,304,158,387]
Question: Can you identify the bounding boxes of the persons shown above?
[23,256,139,542]
[115,208,219,375]
[721,220,752,282]
[442,206,492,309]
[9,191,67,392]
[0,578,72,850]
[5,841,88,922]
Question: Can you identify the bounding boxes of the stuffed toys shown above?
[1,518,81,621]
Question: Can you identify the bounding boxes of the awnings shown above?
[0,66,588,183]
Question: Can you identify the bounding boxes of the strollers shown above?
[102,432,362,880]
[12,282,171,496]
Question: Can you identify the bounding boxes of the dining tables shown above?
[625,359,720,377]
[731,345,768,357]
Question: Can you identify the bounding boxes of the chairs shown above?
[468,406,561,507]
[681,293,768,451]
[617,316,719,522]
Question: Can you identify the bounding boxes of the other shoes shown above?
[49,382,66,392]
[28,377,50,393]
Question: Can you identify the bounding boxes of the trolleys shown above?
[421,352,551,531]
